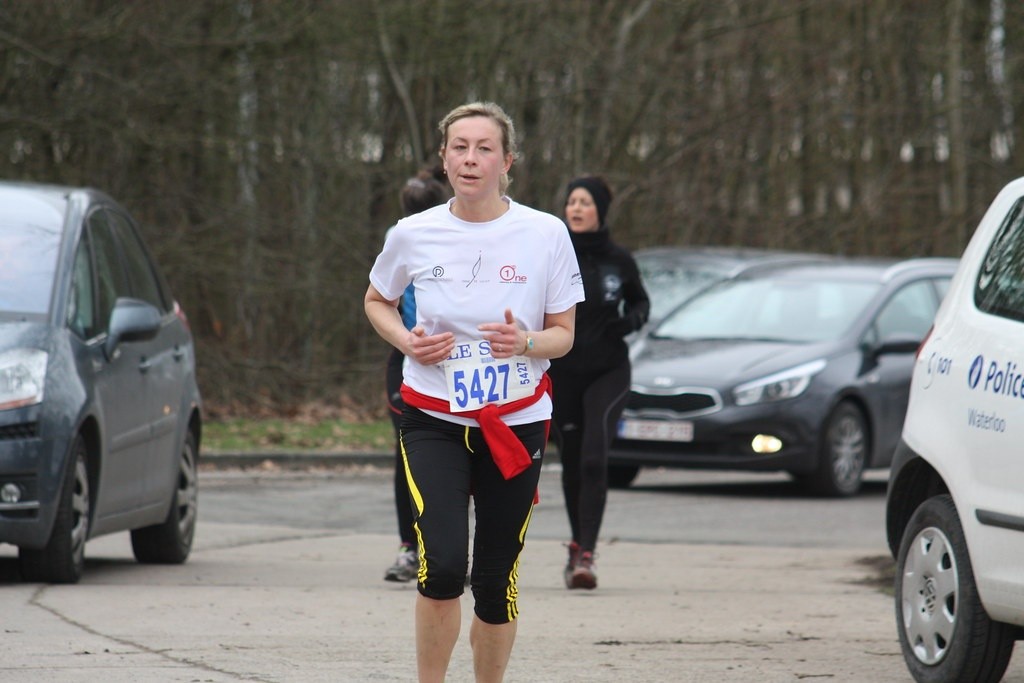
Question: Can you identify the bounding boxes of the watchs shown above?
[516,330,535,356]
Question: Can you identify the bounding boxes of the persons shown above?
[383,165,473,587]
[366,103,586,683]
[545,176,652,588]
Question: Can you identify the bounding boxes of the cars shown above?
[597,253,1023,500]
[884,177,1024,683]
[0,167,206,587]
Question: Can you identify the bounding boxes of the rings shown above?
[499,343,502,351]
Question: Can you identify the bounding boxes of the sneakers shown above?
[565,543,597,589]
[385,545,418,582]
